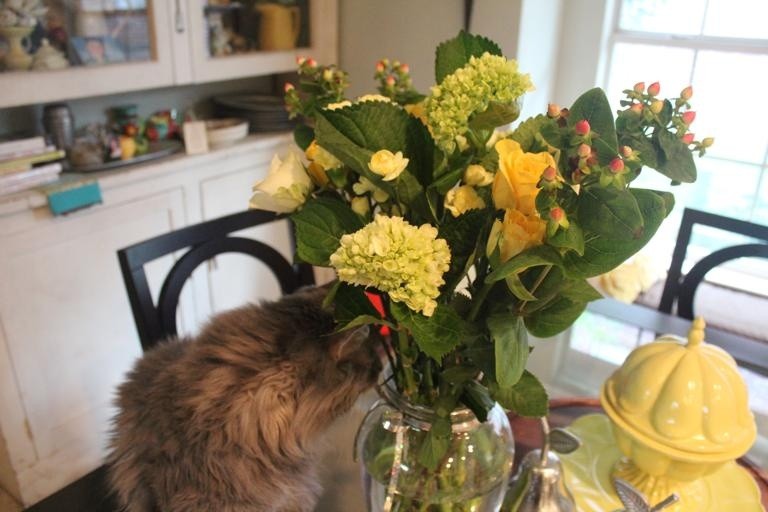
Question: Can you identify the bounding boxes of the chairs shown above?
[651,209,765,348]
[116,206,317,352]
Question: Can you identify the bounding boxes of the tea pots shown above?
[254,2,301,49]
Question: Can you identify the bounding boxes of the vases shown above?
[352,369,515,511]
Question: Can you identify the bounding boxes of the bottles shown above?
[44,103,74,155]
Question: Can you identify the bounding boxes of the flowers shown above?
[251,26,715,511]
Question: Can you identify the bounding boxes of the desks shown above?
[505,293,767,509]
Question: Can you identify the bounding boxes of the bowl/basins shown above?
[213,94,315,131]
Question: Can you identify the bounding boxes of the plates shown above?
[201,119,248,147]
[551,413,765,511]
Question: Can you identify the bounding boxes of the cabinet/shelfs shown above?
[1,1,350,508]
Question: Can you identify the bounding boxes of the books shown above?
[0,135,65,196]
